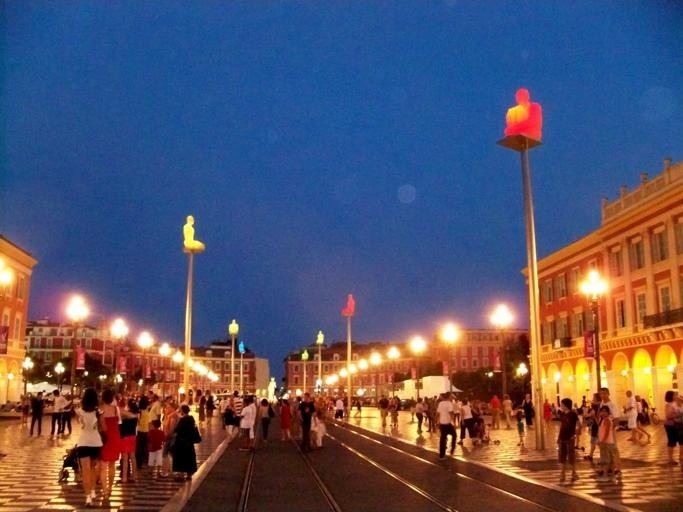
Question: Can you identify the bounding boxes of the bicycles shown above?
[647,407,661,426]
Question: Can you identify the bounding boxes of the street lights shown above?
[578,267,611,392]
[294,290,527,446]
[499,86,548,454]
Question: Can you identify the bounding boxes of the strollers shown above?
[55,443,83,483]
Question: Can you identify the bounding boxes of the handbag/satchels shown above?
[193,426,201,443]
[95,408,107,444]
[267,404,274,418]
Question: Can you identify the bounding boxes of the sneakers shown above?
[559,472,566,481]
[91,490,96,498]
[84,497,92,508]
[583,455,621,476]
[571,475,579,482]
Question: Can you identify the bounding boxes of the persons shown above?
[205,395,215,424]
[47,388,68,434]
[388,399,399,427]
[514,410,525,445]
[197,396,205,424]
[543,397,551,424]
[217,390,348,450]
[410,391,490,462]
[29,391,48,435]
[487,393,502,428]
[500,394,513,427]
[555,385,682,482]
[351,400,360,417]
[521,392,534,426]
[376,394,389,428]
[59,383,202,506]
